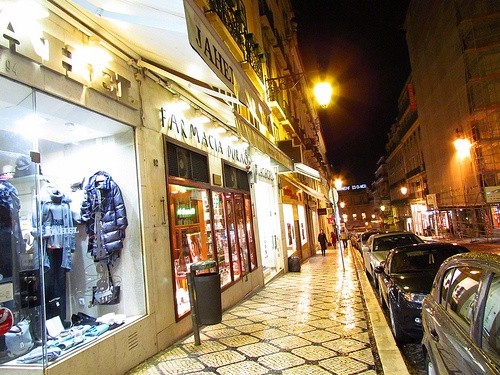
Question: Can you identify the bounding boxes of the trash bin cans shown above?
[190,261,222,325]
[288,256,301,272]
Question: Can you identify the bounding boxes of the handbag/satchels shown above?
[328,242,331,246]
[4,317,35,358]
[92,264,120,305]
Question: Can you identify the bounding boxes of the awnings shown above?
[157,74,294,170]
[71,0,273,124]
[280,173,332,204]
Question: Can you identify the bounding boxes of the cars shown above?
[362,231,424,288]
[372,242,472,339]
[353,227,384,253]
[420,250,500,375]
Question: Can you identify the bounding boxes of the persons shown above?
[32,189,76,318]
[0,165,25,279]
[341,227,348,249]
[318,229,329,256]
[331,232,337,249]
